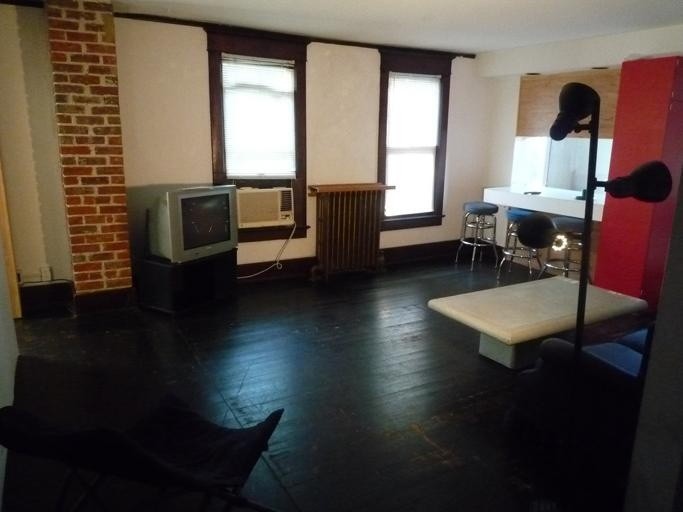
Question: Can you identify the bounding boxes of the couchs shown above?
[539,321,655,402]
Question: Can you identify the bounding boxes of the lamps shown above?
[548,82,673,356]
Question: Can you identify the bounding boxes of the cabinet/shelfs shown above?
[134,250,238,317]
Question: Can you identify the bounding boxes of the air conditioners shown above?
[237,186,296,228]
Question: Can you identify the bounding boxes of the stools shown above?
[497,208,543,280]
[537,216,594,279]
[455,202,499,272]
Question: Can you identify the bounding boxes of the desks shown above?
[428,275,649,371]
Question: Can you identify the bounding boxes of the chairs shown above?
[1,391,283,512]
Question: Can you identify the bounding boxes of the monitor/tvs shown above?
[145,184,239,263]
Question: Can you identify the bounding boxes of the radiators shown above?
[300,182,397,284]
